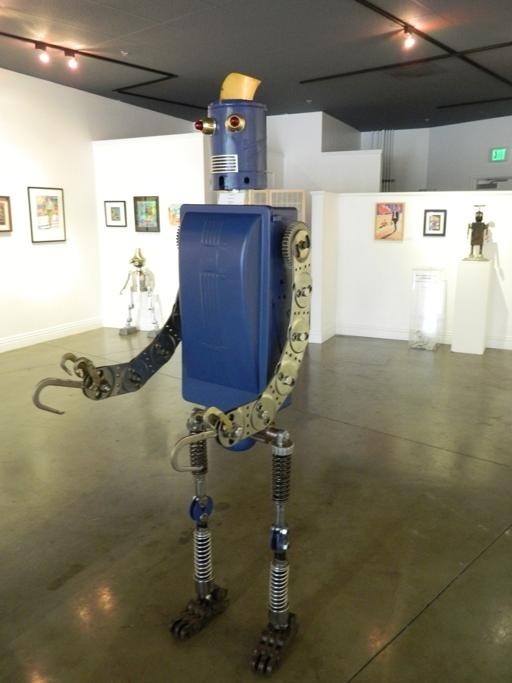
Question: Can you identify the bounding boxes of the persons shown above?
[463,211,490,258]
[391,206,400,231]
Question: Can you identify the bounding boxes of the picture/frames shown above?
[374,201,405,242]
[104,201,127,227]
[27,186,66,243]
[0,196,13,232]
[134,196,160,232]
[424,209,447,236]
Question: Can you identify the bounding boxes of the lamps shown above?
[402,23,415,49]
[34,39,79,70]
